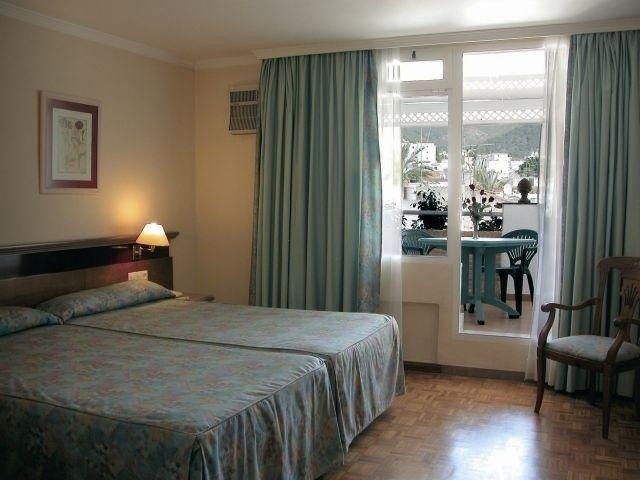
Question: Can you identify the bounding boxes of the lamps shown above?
[132,223,170,261]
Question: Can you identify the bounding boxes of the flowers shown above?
[463,184,494,230]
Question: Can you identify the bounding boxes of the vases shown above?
[474,225,479,238]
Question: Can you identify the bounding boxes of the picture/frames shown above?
[40,91,101,195]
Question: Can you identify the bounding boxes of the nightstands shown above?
[175,293,215,301]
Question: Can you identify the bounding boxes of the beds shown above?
[35,298,406,454]
[0,325,344,480]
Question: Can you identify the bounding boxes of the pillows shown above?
[39,279,176,322]
[0,304,64,335]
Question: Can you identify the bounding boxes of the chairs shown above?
[534,257,640,439]
[468,230,538,313]
[401,229,436,255]
